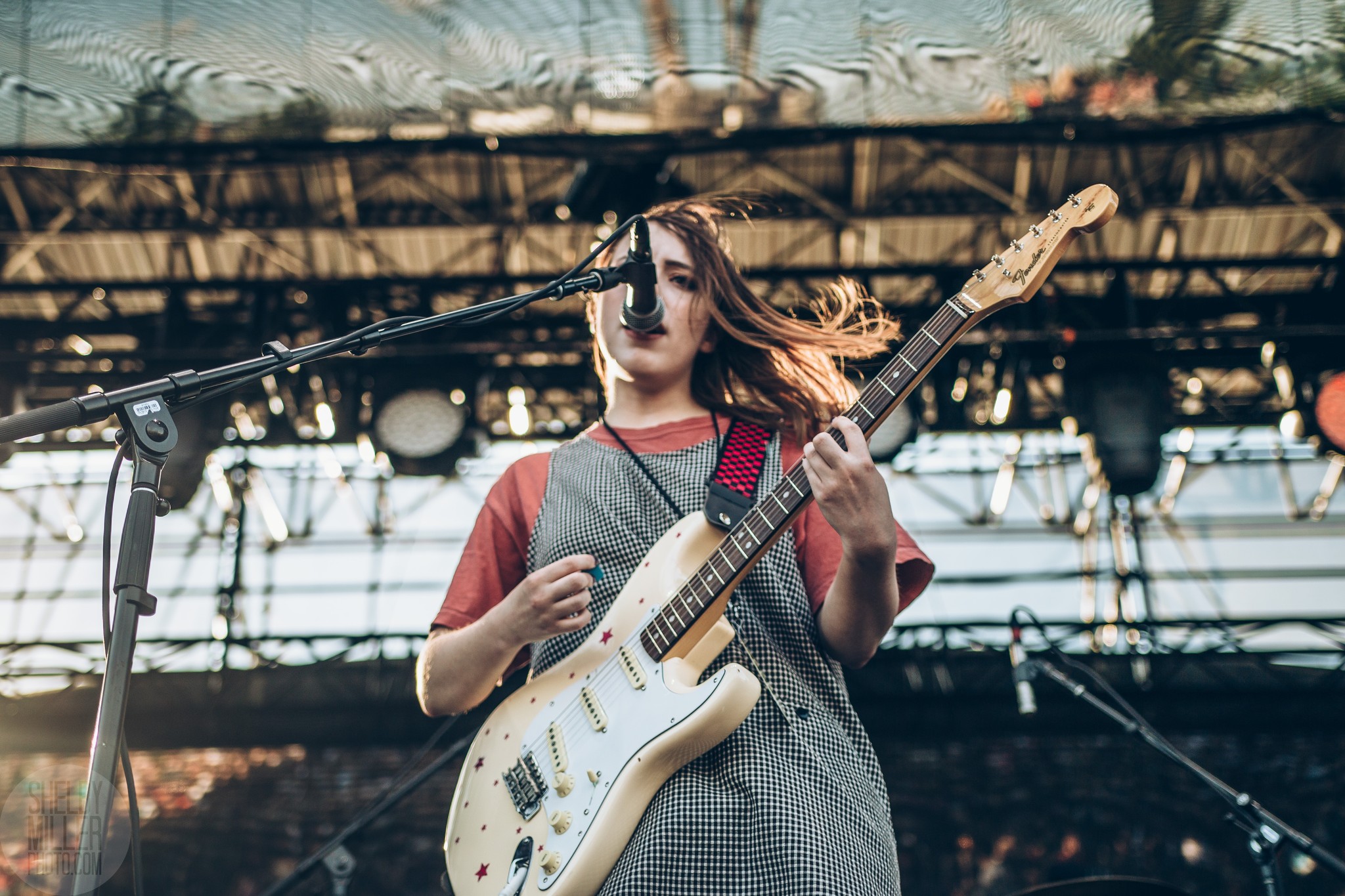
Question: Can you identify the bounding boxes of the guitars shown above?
[441,183,1119,896]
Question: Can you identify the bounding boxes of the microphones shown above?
[618,217,667,333]
[1010,614,1038,715]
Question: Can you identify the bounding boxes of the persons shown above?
[415,198,935,896]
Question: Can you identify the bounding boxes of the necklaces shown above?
[597,408,724,521]
